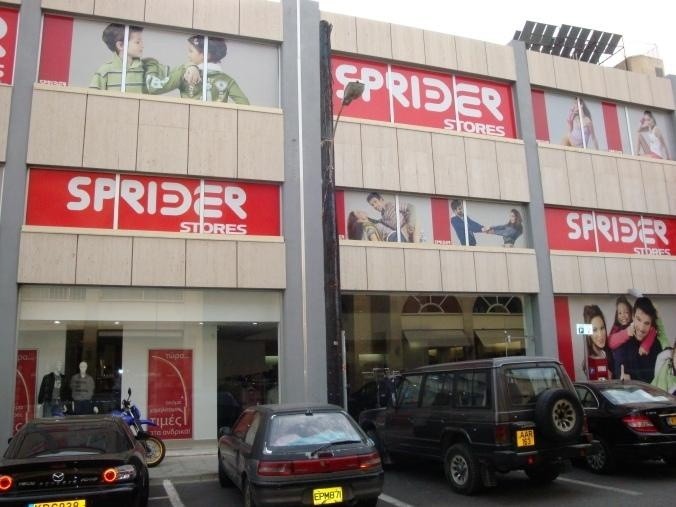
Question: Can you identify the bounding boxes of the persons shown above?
[581,289,676,397]
[70,360,96,414]
[449,198,490,246]
[89,22,203,95]
[634,110,672,160]
[487,208,524,247]
[142,34,250,105]
[347,210,423,243]
[38,360,70,417]
[367,192,427,242]
[561,97,599,150]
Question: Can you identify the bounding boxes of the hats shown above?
[187,33,228,63]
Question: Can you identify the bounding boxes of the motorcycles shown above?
[52,385,166,469]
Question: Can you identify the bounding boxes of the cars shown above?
[216,403,385,507]
[0,413,149,506]
[568,379,676,472]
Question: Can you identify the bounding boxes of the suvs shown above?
[358,356,586,493]
